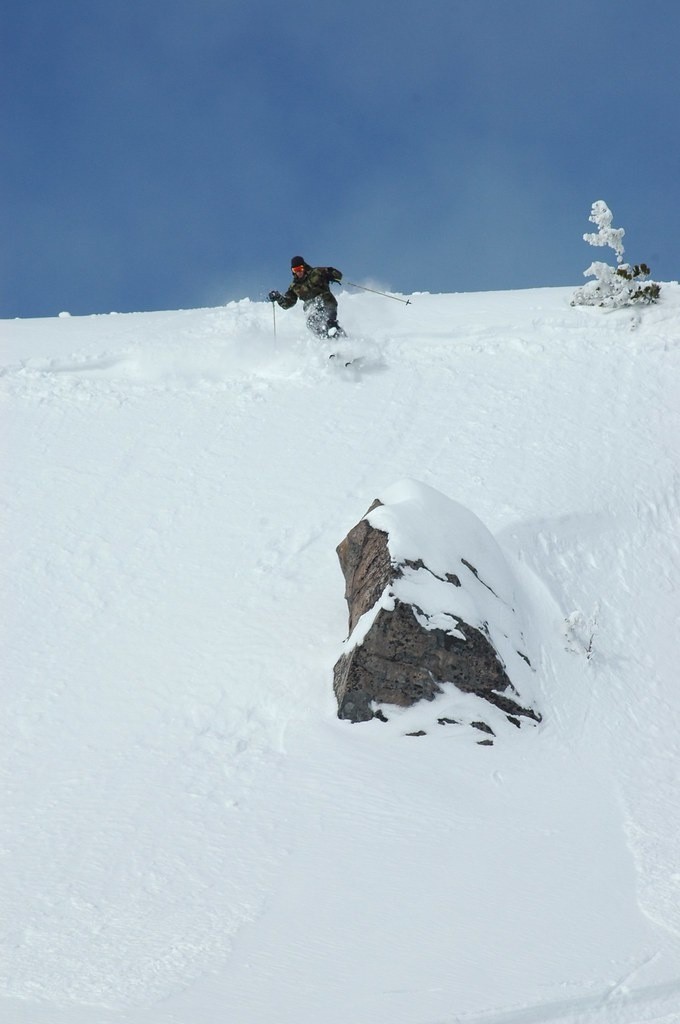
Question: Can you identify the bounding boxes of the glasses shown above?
[292,265,304,273]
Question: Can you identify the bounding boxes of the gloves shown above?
[269,291,280,302]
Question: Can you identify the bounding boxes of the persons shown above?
[269,256,348,340]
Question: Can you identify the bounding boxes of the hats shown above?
[291,255,305,267]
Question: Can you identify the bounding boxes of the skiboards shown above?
[327,354,360,368]
